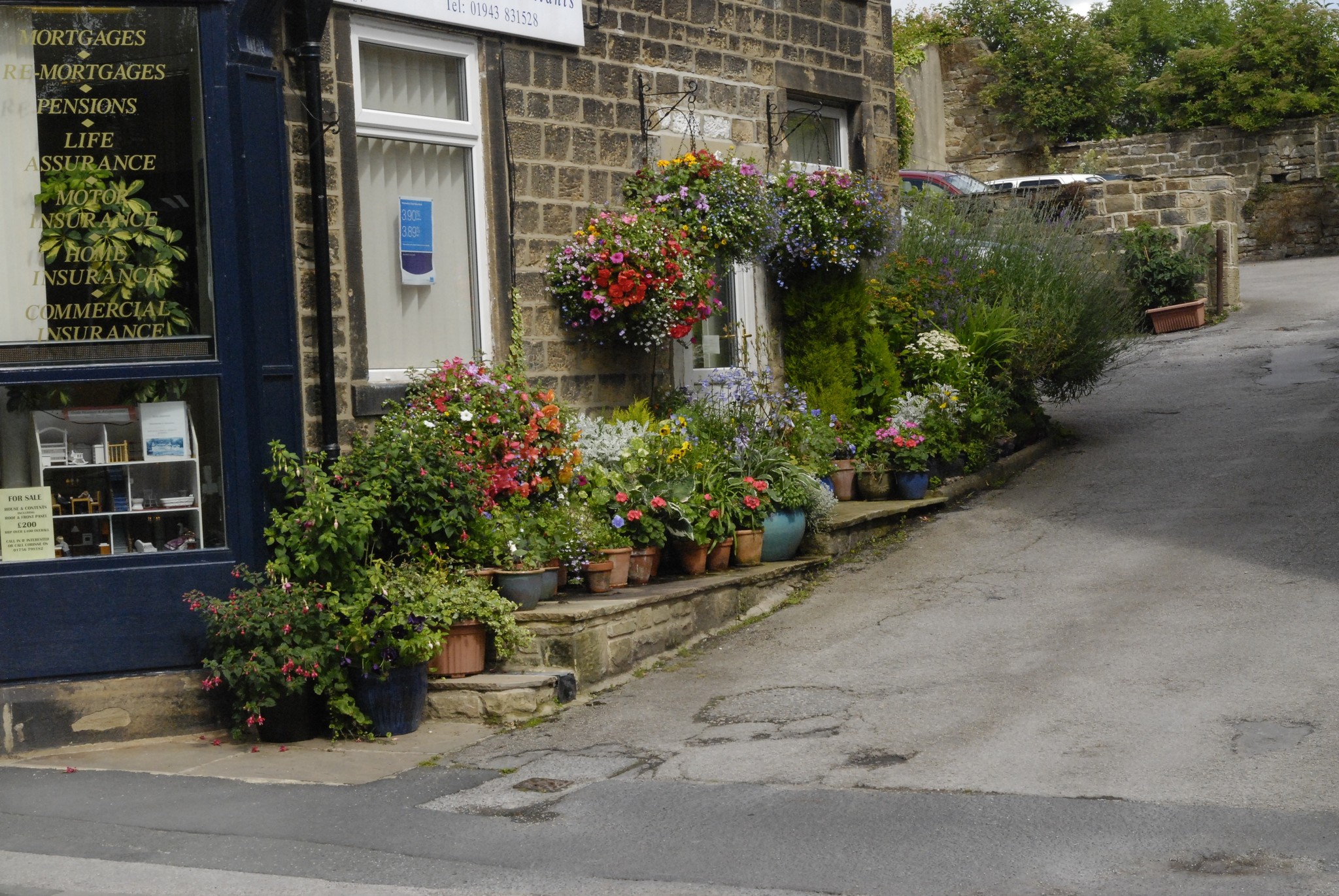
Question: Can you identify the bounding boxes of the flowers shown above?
[185,147,1160,753]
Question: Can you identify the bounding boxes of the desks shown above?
[72,498,91,514]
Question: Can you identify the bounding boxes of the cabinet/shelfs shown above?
[92,444,104,464]
[42,454,51,466]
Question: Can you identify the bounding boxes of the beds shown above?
[38,426,69,465]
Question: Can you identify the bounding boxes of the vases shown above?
[454,567,495,594]
[896,467,930,500]
[856,467,894,502]
[707,536,735,571]
[629,545,657,586]
[827,456,862,500]
[549,557,567,586]
[357,650,430,739]
[597,546,633,587]
[539,566,561,601]
[679,539,709,575]
[496,568,546,612]
[731,527,765,567]
[580,560,613,593]
[762,501,806,560]
[255,685,318,743]
[649,547,663,577]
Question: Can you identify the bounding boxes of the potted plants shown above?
[388,563,538,679]
[1108,221,1214,334]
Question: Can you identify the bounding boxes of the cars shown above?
[900,165,1143,196]
[899,203,1045,282]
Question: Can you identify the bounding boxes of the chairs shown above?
[142,488,160,508]
[51,493,62,515]
[90,490,102,512]
